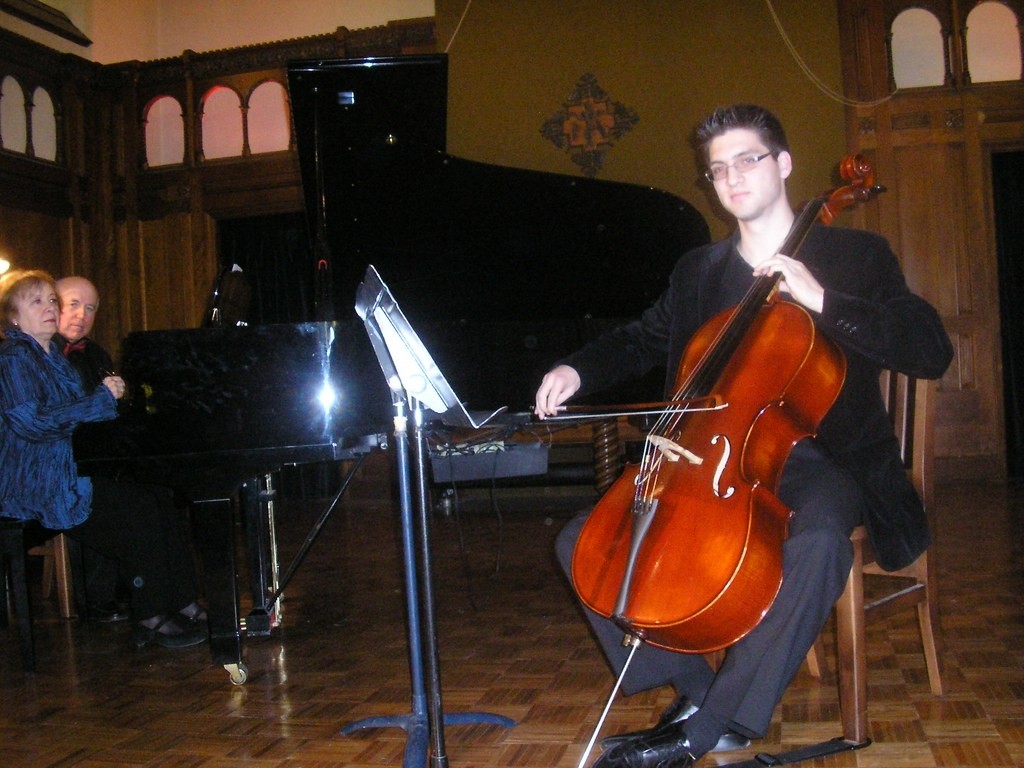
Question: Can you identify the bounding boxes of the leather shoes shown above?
[599,690,748,752]
[591,731,699,768]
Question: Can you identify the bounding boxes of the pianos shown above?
[67,310,397,668]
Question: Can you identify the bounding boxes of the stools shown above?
[25,532,78,619]
[0,517,88,673]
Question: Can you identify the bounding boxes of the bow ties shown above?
[64,342,87,354]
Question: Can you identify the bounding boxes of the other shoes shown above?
[170,602,209,629]
[133,620,205,649]
[78,602,128,623]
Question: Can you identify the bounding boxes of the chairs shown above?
[704,368,951,745]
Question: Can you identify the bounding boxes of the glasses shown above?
[706,151,776,180]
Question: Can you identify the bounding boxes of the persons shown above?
[535,103,954,768]
[49,276,130,622]
[1,272,208,648]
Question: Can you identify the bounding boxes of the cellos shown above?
[567,147,890,662]
